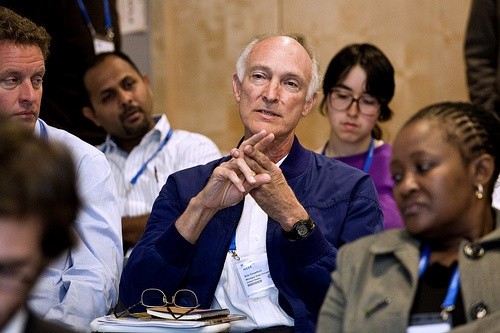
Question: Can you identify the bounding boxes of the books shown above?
[94,305,248,329]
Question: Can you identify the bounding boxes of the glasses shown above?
[112,288,200,320]
[330,89,384,117]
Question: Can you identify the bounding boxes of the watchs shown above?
[280,215,314,240]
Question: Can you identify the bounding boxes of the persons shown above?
[118,34,383,333]
[0,6,124,333]
[315,100,500,333]
[77,51,223,274]
[307,42,409,231]
[0,114,81,333]
